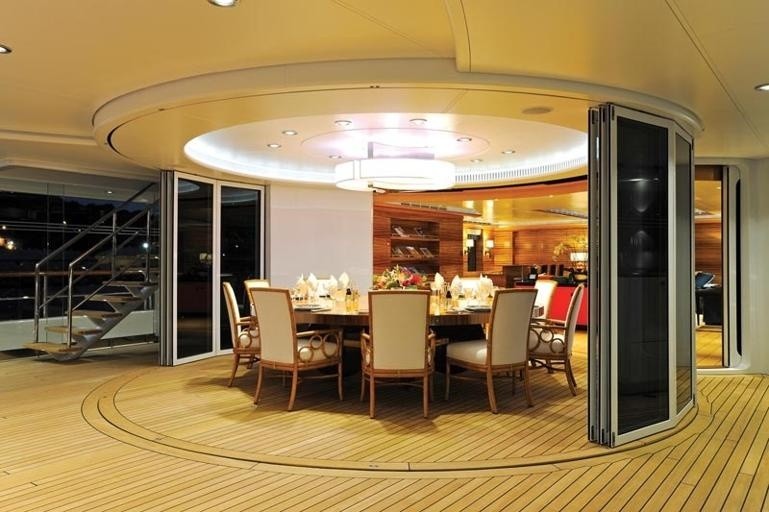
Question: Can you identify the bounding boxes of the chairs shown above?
[223,282,287,392]
[509,282,584,398]
[517,279,559,374]
[248,285,345,411]
[446,287,533,415]
[245,279,272,314]
[359,289,437,417]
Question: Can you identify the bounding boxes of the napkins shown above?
[292,273,495,296]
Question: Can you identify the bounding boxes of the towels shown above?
[434,272,496,298]
[296,272,349,296]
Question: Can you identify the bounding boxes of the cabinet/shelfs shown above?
[391,234,440,262]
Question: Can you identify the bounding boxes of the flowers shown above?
[373,264,428,287]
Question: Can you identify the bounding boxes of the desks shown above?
[263,292,548,414]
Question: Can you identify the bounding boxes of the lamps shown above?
[334,142,455,191]
